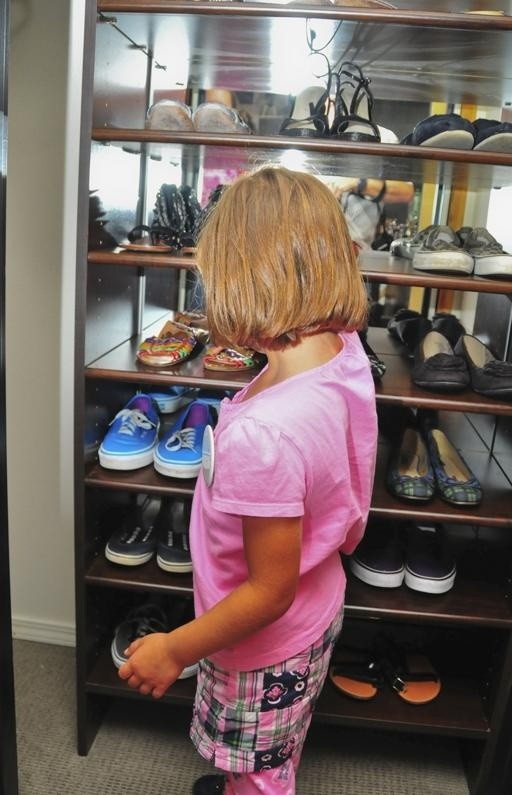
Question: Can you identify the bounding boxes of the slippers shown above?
[137,321,264,371]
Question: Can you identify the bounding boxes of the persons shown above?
[115,163,383,795]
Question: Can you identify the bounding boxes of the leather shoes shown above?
[412,330,512,395]
[387,308,466,345]
[388,428,482,505]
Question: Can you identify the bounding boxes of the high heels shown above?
[326,61,380,144]
[280,52,331,138]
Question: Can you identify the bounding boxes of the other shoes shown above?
[400,114,475,150]
[145,100,195,132]
[192,102,256,135]
[469,117,512,153]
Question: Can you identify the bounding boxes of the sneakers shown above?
[412,225,512,275]
[98,394,212,479]
[349,521,457,594]
[105,496,193,573]
[390,225,434,258]
[192,775,225,795]
[148,386,200,414]
[112,619,199,680]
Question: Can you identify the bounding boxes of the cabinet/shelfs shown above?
[72,1,510,791]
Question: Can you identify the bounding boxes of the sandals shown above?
[329,646,441,705]
[120,184,224,253]
